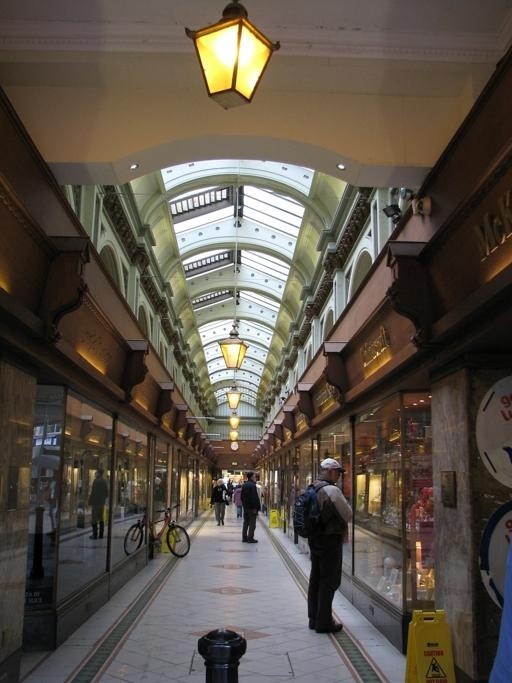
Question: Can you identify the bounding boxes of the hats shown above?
[320,458,345,472]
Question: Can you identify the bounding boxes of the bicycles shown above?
[123,512,147,557]
[148,502,192,559]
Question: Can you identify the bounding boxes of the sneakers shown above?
[89,534,103,539]
[243,538,257,543]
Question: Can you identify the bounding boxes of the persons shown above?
[211,476,265,511]
[153,476,166,520]
[302,456,352,632]
[210,478,226,526]
[240,471,260,543]
[233,484,243,518]
[88,468,108,539]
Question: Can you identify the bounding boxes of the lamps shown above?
[185,0,280,110]
[217,325,249,452]
[382,204,403,223]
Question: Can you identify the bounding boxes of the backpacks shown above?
[292,482,334,538]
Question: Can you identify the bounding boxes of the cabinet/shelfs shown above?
[353,389,433,618]
